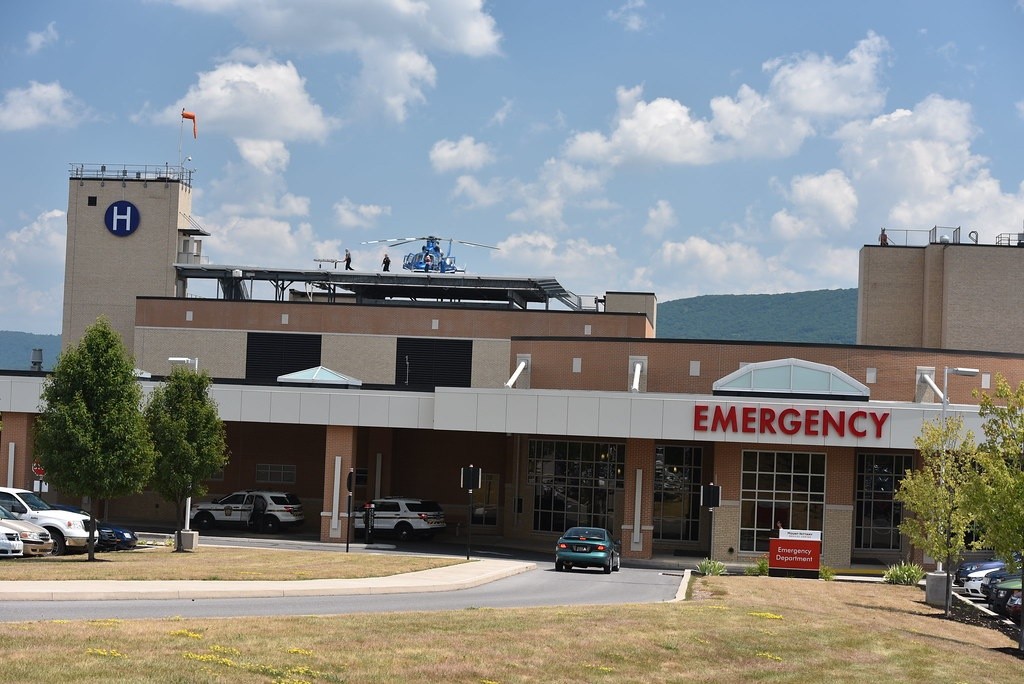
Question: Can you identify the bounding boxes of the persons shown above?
[382,254,391,272]
[344,248,354,271]
[879,230,889,246]
[438,258,447,273]
[424,253,431,273]
[775,520,784,537]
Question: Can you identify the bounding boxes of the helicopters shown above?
[360,234,501,274]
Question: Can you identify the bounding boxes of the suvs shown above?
[0,486,100,556]
[352,495,447,543]
[185,488,305,535]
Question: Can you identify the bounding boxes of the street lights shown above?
[937,364,980,574]
[167,356,199,532]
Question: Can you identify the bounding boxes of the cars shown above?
[955,550,1023,629]
[0,504,55,559]
[0,525,24,559]
[48,503,138,552]
[555,526,621,574]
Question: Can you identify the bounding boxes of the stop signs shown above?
[32,458,48,476]
